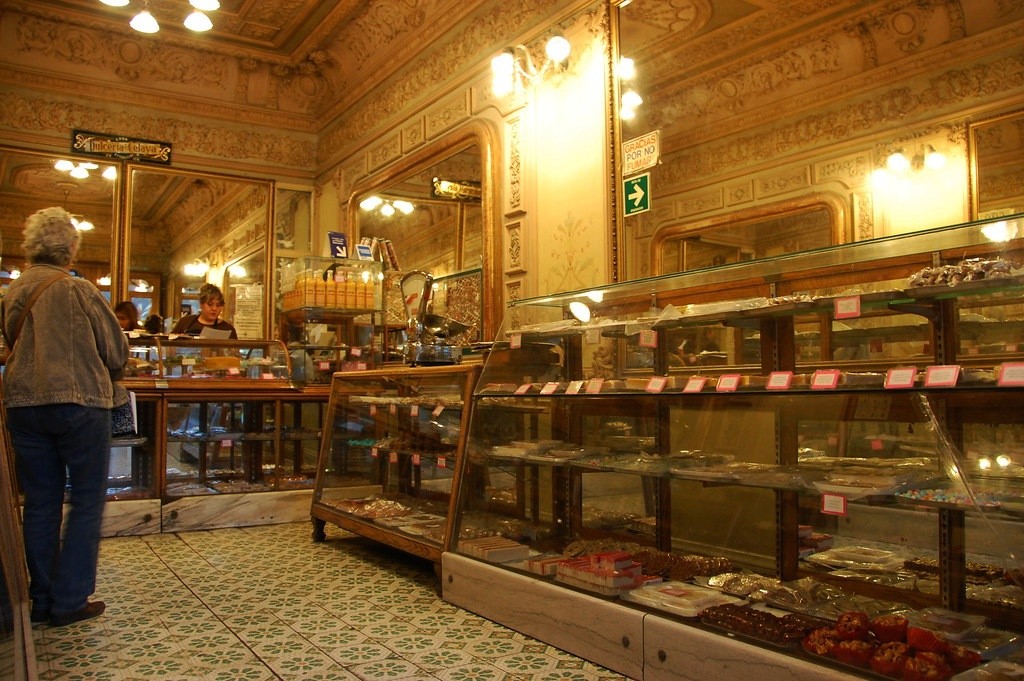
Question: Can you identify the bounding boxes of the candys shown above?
[896,488,1024,507]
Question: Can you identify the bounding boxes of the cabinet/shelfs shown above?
[441,212,1024,681]
[278,307,387,477]
[0,392,162,541]
[162,390,384,532]
[309,365,569,584]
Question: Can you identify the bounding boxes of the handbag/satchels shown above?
[110,390,137,438]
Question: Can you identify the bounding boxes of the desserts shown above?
[457,537,1024,681]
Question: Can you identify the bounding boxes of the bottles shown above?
[284,269,375,310]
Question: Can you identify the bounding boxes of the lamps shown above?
[97,0,220,33]
[360,196,414,217]
[54,160,98,178]
[55,182,95,230]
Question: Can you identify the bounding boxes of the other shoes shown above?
[52,601,105,628]
[30,610,52,623]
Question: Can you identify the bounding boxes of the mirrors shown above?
[649,189,847,278]
[118,162,276,345]
[345,119,502,354]
[604,0,1024,522]
[965,107,1024,224]
[0,143,122,315]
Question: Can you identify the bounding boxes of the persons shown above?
[115,283,238,340]
[0,208,129,626]
